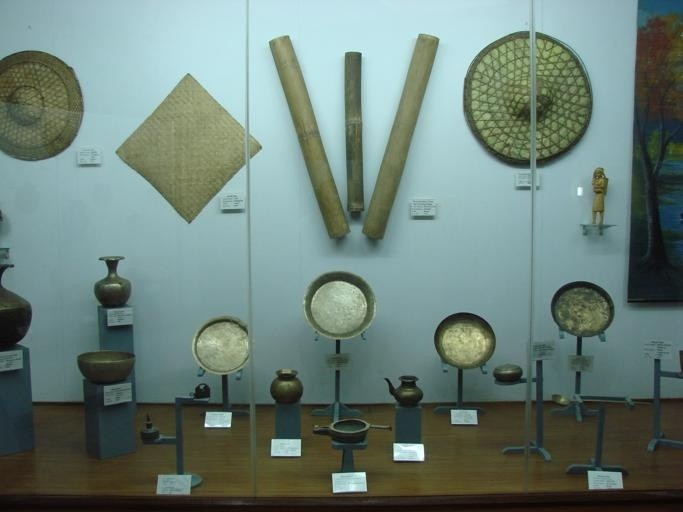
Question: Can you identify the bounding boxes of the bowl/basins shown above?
[493,363,524,382]
[77,350,136,384]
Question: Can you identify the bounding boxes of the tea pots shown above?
[383,376,424,408]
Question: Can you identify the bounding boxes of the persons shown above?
[590,167,608,225]
